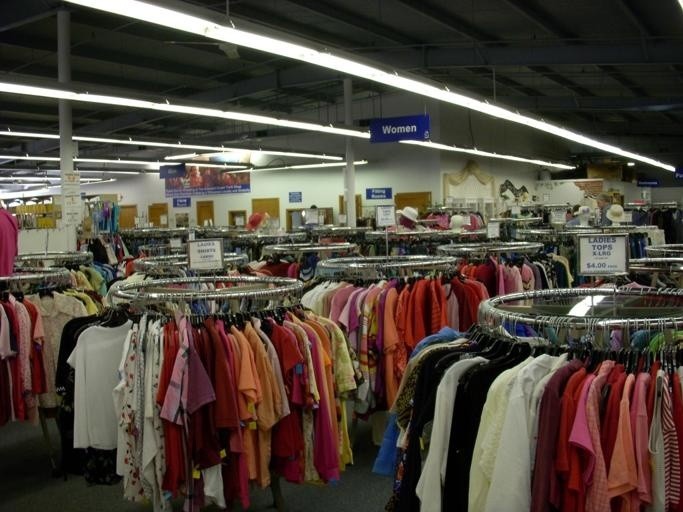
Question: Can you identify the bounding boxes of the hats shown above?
[398,206,419,223]
[573,206,594,215]
[597,193,609,199]
[605,204,625,222]
[248,212,266,229]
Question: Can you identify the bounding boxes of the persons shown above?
[168,166,250,190]
[566,204,581,225]
[448,214,465,234]
[576,207,595,225]
[246,211,271,234]
[597,193,613,226]
[606,204,625,226]
[395,206,418,232]
